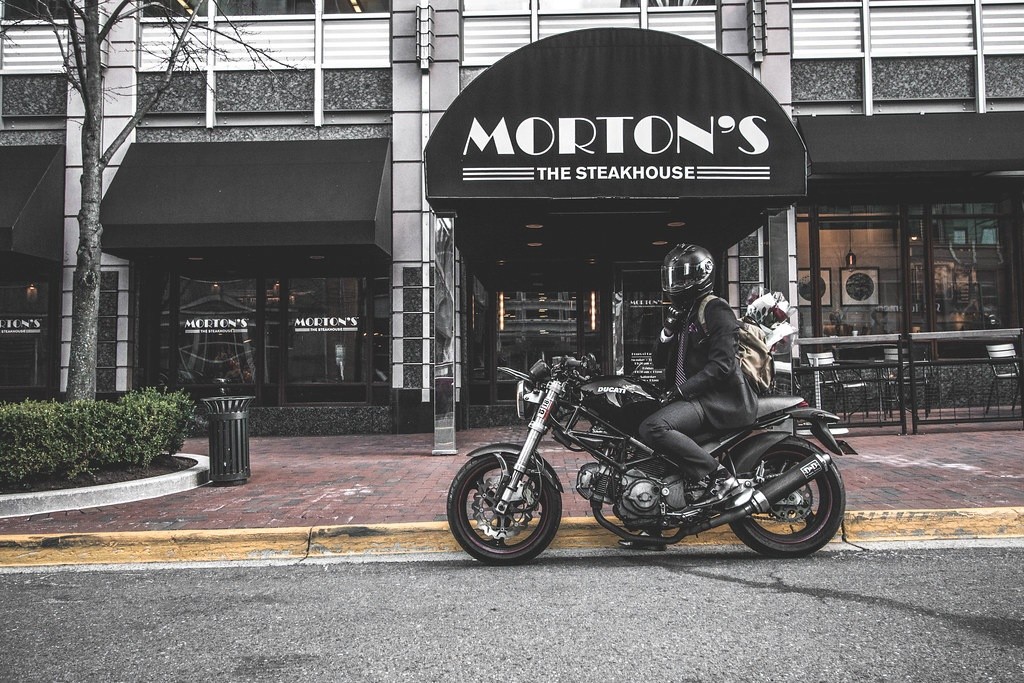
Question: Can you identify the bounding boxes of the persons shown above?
[830,309,850,336]
[870,308,888,334]
[618,242,758,550]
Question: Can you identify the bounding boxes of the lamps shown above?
[846,229,858,267]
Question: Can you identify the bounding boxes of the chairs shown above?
[884,347,932,420]
[985,344,1019,413]
[807,352,869,421]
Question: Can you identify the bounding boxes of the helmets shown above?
[660,243,716,306]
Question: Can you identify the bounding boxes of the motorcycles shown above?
[446,348,859,569]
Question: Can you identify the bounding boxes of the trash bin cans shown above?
[200,395,255,486]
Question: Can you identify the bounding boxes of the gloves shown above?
[664,288,695,334]
[658,392,681,409]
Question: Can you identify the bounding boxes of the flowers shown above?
[736,284,800,394]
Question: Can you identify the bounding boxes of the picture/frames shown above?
[839,267,880,306]
[797,267,832,307]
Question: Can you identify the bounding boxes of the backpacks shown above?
[698,294,776,397]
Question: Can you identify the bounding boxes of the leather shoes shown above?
[693,467,739,506]
[618,530,666,551]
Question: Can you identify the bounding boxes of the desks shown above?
[799,328,1024,359]
[833,359,899,422]
